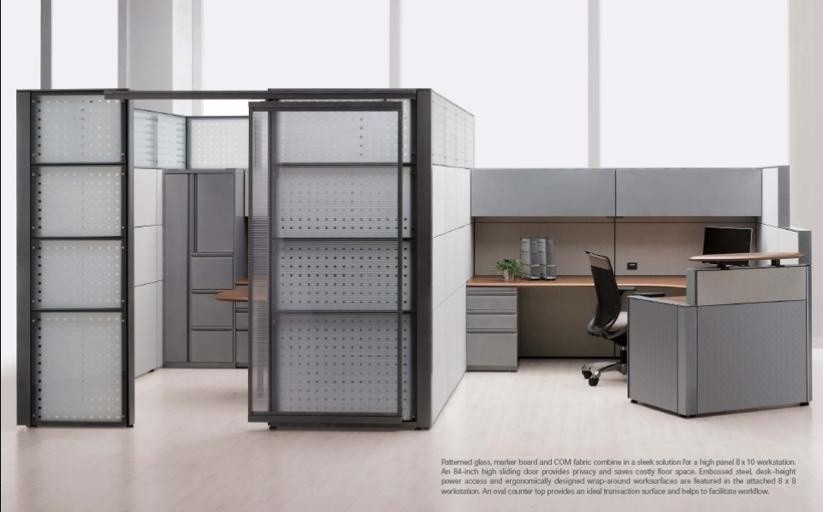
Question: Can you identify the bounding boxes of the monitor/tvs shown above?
[700,225,752,266]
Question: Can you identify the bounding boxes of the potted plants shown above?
[495,257,523,282]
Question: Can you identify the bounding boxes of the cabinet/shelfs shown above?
[235,284,249,369]
[626,252,812,420]
[161,167,244,368]
[465,287,518,372]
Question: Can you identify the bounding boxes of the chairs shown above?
[582,248,665,387]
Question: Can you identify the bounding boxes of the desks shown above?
[465,276,686,372]
